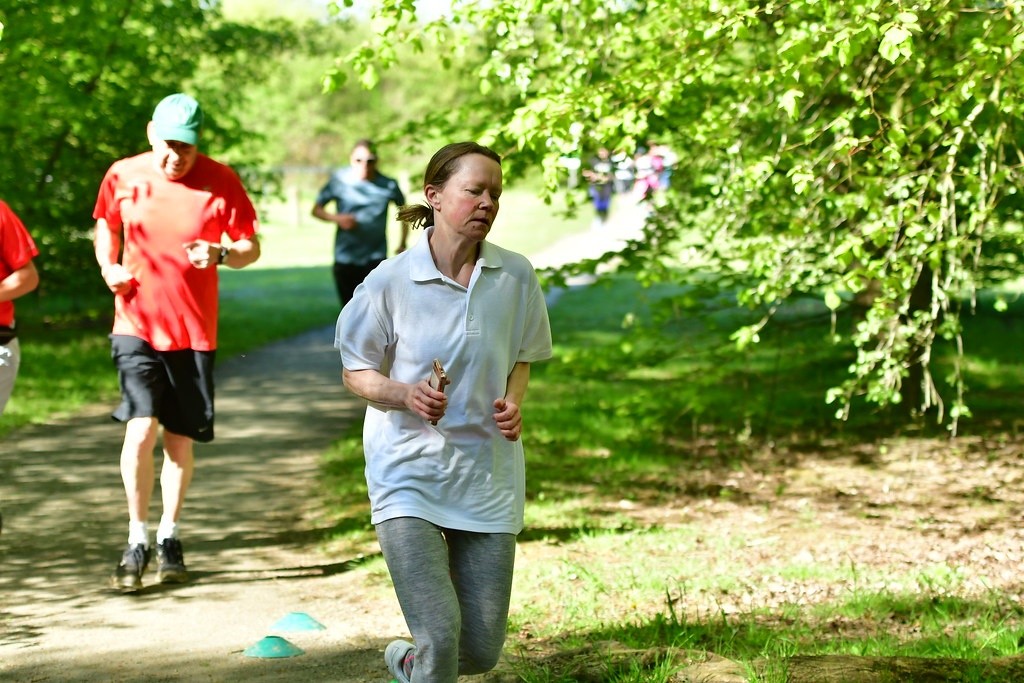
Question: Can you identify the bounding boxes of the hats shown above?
[151,93,204,146]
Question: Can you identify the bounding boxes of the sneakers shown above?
[156,538,189,583]
[383,640,417,683]
[112,544,151,590]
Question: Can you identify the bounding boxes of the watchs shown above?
[218,246,229,264]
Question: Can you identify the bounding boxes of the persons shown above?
[583,138,678,224]
[333,142,553,683]
[0,198,41,415]
[310,140,409,307]
[91,95,261,594]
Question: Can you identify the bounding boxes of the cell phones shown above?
[428,358,445,426]
